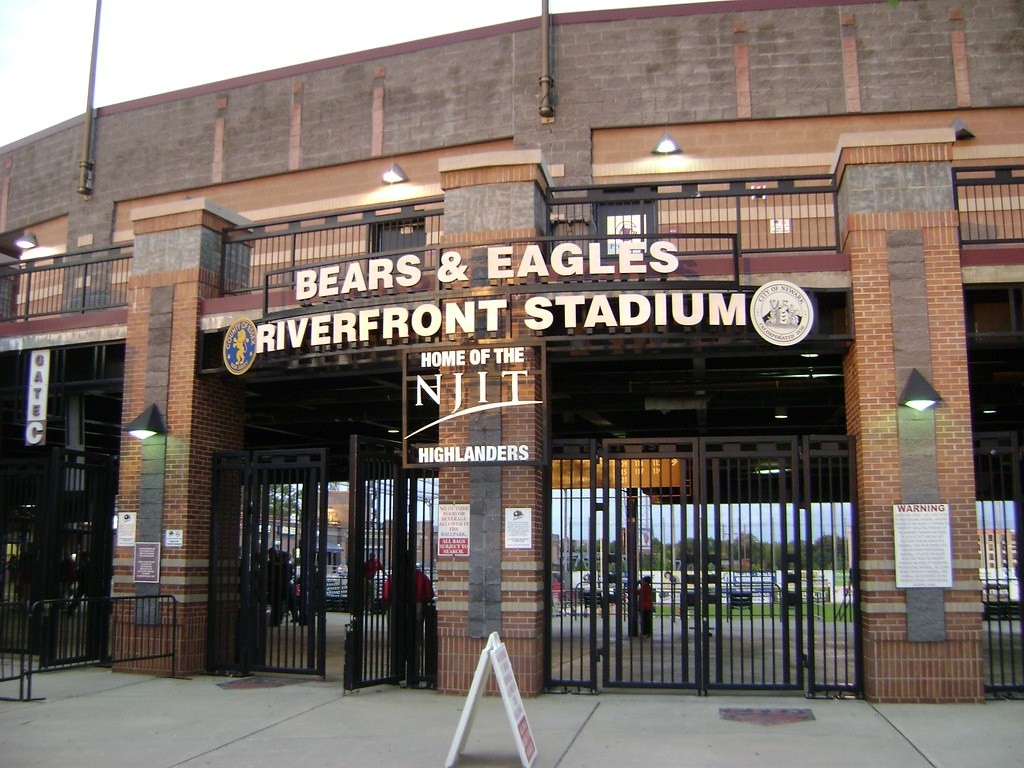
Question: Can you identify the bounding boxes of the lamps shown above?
[15,233,38,250]
[951,117,976,140]
[382,162,408,184]
[122,403,164,440]
[897,368,943,411]
[651,133,680,154]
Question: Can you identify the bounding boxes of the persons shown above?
[633,576,653,637]
[267,548,434,689]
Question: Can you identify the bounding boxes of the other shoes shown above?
[291,618,299,622]
[642,634,650,638]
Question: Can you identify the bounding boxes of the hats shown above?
[643,576,653,584]
